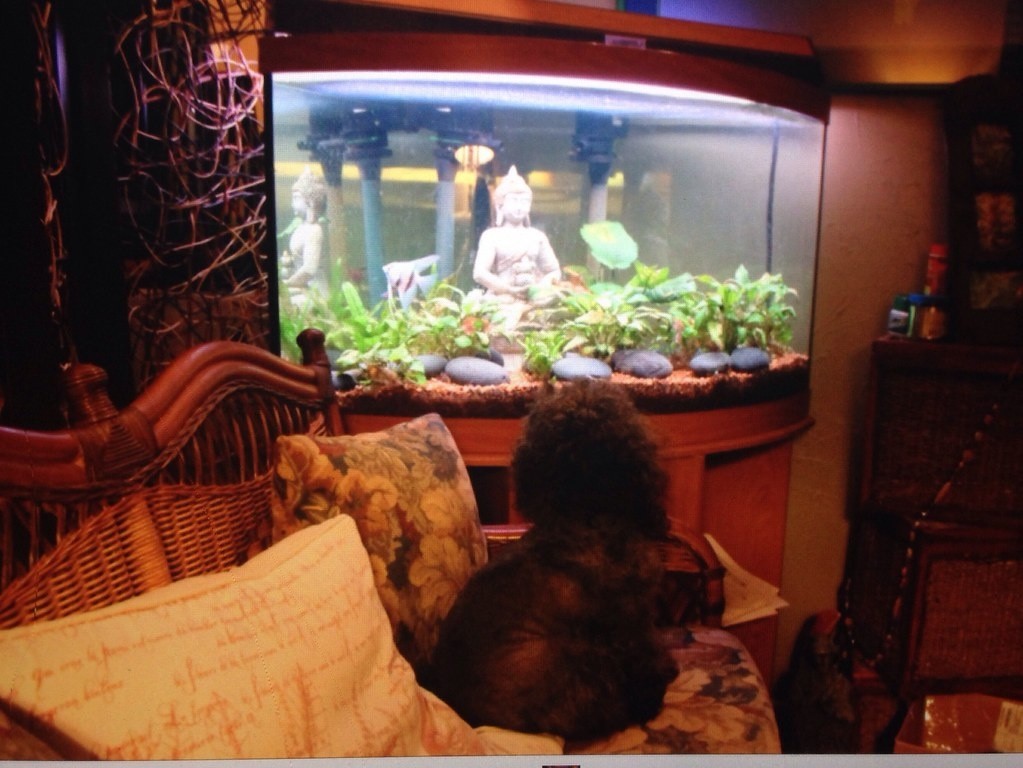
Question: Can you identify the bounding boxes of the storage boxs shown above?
[857,340,1023,519]
[859,510,1023,700]
[266,71,829,412]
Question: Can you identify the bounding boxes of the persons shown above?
[281,166,330,308]
[466,164,563,311]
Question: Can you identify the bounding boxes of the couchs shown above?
[0,318,789,761]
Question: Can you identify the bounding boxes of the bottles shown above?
[885,244,953,339]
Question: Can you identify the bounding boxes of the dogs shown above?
[423,375,706,734]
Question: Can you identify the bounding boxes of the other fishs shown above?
[382,254,439,312]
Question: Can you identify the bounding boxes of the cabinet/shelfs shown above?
[946,91,1023,332]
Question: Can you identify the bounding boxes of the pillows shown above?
[0,516,567,760]
[260,407,495,662]
